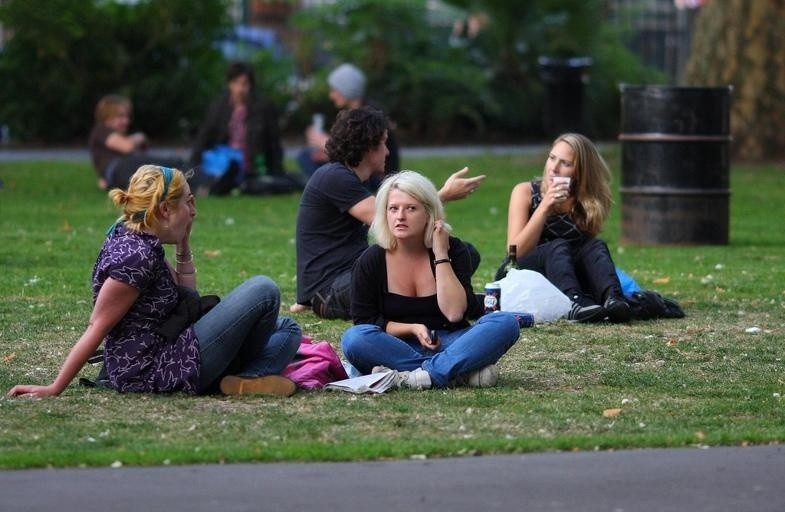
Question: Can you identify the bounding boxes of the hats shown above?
[328,64,368,100]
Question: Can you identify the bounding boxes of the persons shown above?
[290,106,488,319]
[179,61,297,194]
[297,63,401,192]
[87,94,192,190]
[8,163,303,399]
[495,132,631,324]
[342,169,521,391]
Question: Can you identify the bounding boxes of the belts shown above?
[313,286,330,314]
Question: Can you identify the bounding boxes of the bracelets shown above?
[175,265,200,279]
[173,250,197,264]
[432,257,453,266]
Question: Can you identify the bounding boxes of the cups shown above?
[553,177,571,200]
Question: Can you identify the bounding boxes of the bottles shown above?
[504,245,520,277]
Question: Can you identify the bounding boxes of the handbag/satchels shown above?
[625,290,684,318]
[281,336,348,391]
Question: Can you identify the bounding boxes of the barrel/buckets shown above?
[618,83,732,248]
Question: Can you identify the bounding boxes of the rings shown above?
[554,191,562,199]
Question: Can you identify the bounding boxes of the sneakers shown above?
[603,285,632,324]
[559,288,602,320]
[469,365,500,388]
[219,375,297,398]
[371,365,416,392]
